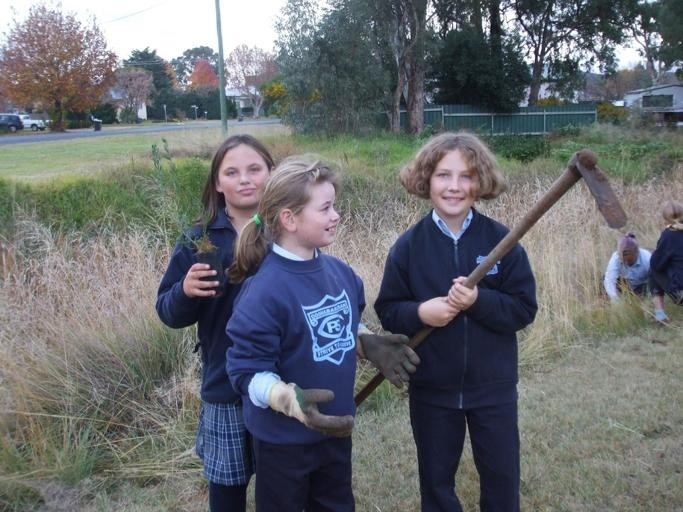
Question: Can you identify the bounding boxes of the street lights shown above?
[192,104,199,119]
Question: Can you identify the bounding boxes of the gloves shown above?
[269,381,354,439]
[356,327,421,389]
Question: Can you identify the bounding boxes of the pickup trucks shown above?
[20,115,46,131]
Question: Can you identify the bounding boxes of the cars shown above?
[0,115,24,132]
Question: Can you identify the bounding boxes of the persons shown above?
[646,198,683,324]
[152,133,279,512]
[223,157,422,512]
[373,128,539,510]
[603,229,653,300]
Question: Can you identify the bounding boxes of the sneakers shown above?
[655,310,667,321]
